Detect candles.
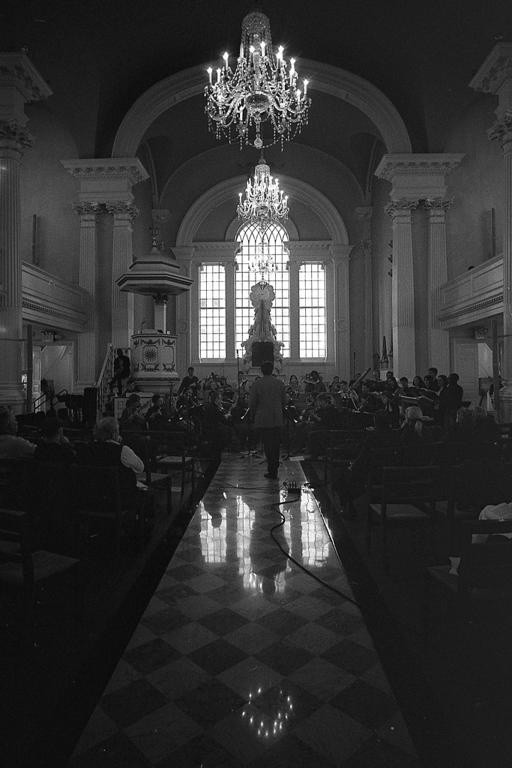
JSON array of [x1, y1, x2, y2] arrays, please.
[[235, 164, 290, 231]]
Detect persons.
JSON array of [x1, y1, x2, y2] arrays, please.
[[34, 416, 77, 469], [117, 363, 464, 478], [0, 403, 38, 458], [79, 415, 148, 492], [446, 485, 511, 584], [108, 348, 130, 393]]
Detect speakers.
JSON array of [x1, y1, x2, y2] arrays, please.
[[252, 344, 272, 367], [83, 386, 96, 426]]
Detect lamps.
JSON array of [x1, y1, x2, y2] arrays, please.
[[205, 12, 315, 151]]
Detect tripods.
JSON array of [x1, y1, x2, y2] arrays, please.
[[281, 418, 292, 461], [238, 415, 262, 459]]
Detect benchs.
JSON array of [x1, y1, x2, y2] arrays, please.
[[1, 419, 197, 732], [308, 425, 512, 682]]
